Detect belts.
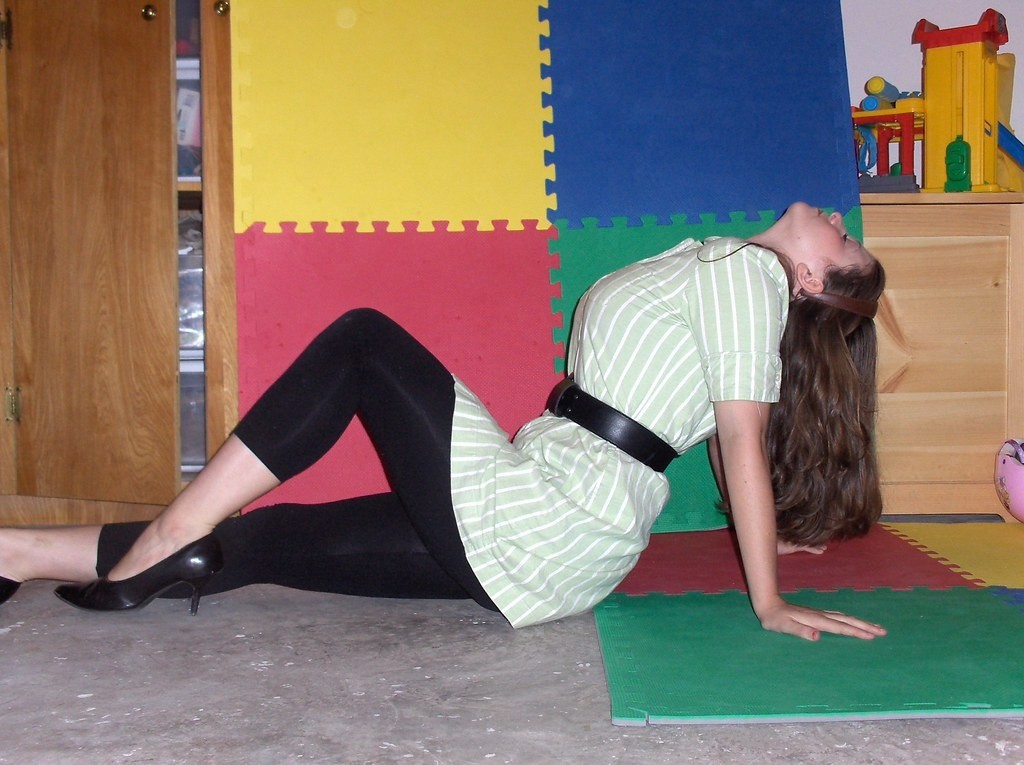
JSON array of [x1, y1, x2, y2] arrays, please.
[[548, 378, 681, 473]]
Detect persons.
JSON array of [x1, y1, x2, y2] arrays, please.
[[0, 200, 886, 640]]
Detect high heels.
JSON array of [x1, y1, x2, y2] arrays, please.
[[0, 576, 23, 605], [53, 533, 224, 616]]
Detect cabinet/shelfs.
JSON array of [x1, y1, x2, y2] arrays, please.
[[861, 192, 1024, 516]]
